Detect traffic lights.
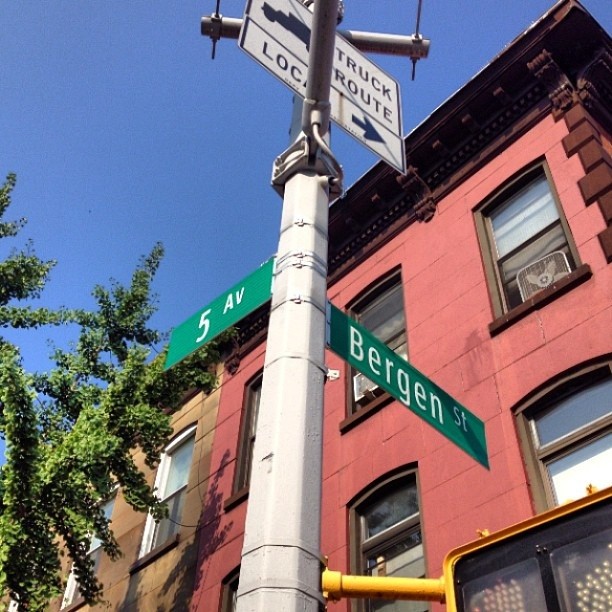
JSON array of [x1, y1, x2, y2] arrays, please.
[[319, 488, 612, 612]]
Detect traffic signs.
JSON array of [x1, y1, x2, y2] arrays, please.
[[163, 256, 274, 375], [237, 0, 409, 175], [325, 298, 491, 472]]
